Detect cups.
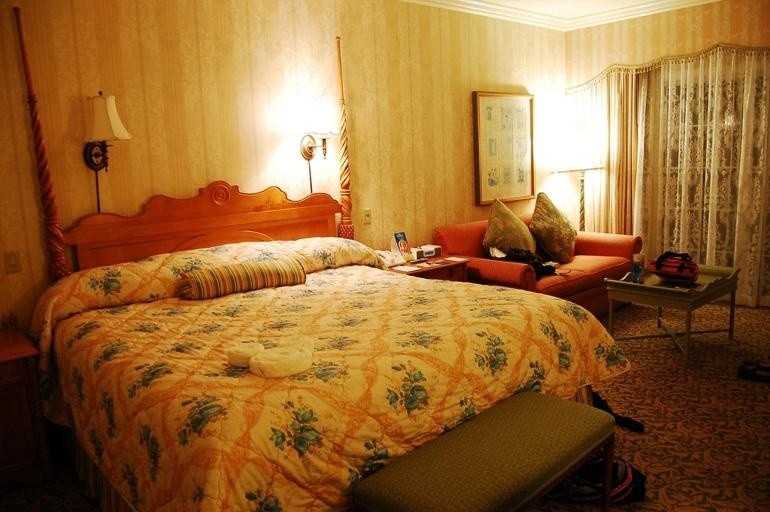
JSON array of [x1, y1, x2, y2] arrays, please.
[[632, 254, 646, 284]]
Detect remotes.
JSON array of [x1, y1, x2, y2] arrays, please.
[[410, 257, 428, 264]]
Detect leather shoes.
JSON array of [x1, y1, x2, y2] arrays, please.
[[738, 366, 770, 381], [744, 360, 770, 371]]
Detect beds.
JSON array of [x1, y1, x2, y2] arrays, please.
[[29, 178, 595, 511]]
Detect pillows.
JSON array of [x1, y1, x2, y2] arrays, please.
[[482, 191, 580, 265]]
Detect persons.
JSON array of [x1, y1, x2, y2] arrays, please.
[[399, 240, 410, 255]]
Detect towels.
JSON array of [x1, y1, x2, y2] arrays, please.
[[226, 334, 316, 379]]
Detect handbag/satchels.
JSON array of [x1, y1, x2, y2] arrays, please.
[[646, 251, 699, 286]]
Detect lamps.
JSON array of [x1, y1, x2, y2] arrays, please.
[[82, 89, 132, 173]]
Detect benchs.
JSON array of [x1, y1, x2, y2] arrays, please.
[[348, 388, 617, 511]]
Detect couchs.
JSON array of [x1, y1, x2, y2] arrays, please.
[[431, 212, 642, 317]]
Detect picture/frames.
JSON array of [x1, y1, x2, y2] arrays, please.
[[470, 90, 537, 207]]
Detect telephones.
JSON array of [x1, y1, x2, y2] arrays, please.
[[375, 250, 406, 268]]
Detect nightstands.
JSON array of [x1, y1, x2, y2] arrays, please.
[[383, 255, 471, 283], [0, 328, 50, 492]]
